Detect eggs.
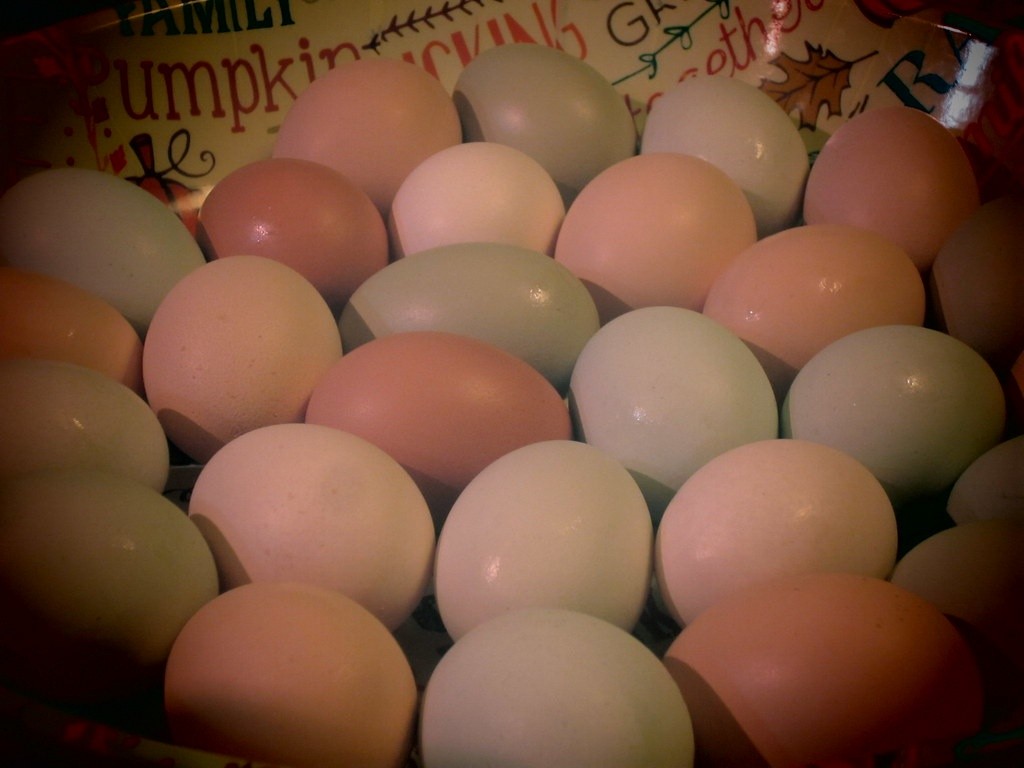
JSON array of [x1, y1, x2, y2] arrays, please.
[[1, 44, 1024, 768]]
